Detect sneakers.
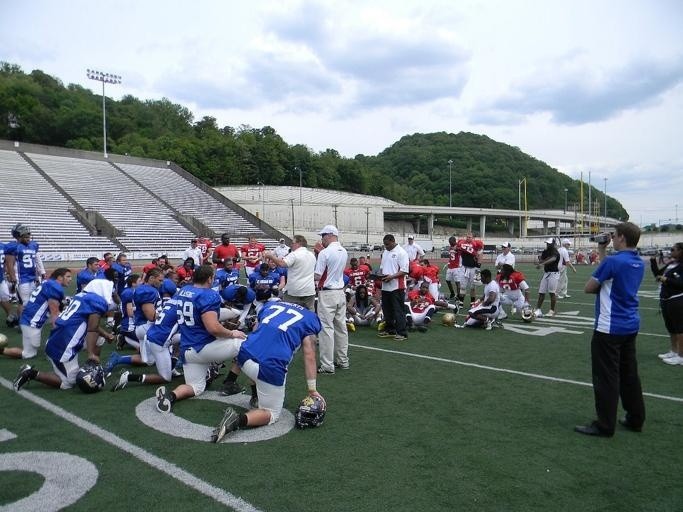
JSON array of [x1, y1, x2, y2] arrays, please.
[[657, 351, 683, 366]]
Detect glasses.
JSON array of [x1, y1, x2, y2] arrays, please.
[[670, 248, 679, 253]]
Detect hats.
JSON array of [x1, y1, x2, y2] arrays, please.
[[562, 239, 571, 244], [501, 242, 511, 248], [408, 236, 414, 239], [318, 225, 338, 236], [544, 238, 555, 245]]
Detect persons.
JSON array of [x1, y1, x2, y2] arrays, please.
[[572, 223, 646, 438], [648, 239, 683, 367], [572, 238, 666, 267], [350, 222, 572, 342], [1, 220, 349, 444]]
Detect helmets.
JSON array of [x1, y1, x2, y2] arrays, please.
[[473, 275, 483, 285], [12, 225, 30, 239]]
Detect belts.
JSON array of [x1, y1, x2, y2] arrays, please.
[[323, 287, 331, 291]]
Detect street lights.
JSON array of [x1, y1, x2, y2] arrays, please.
[[87, 68, 121, 158], [293, 166, 302, 206]]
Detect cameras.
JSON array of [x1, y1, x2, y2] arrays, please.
[[589, 232, 611, 243]]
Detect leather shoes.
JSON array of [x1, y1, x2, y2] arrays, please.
[[618, 419, 642, 433], [574, 425, 615, 437]]
